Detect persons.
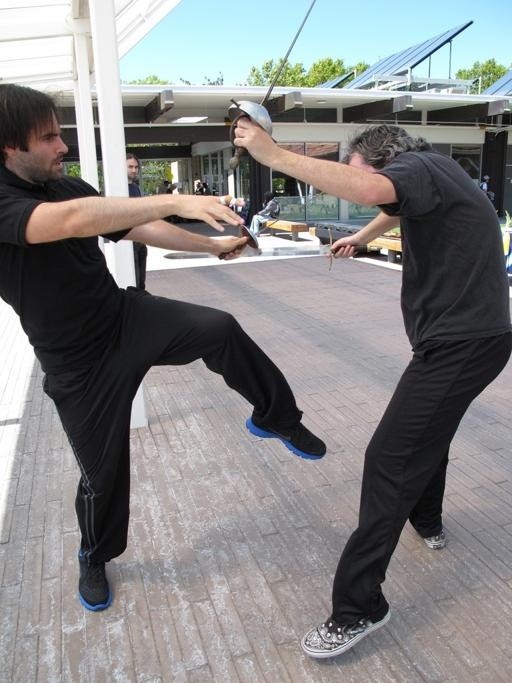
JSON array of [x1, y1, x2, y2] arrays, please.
[[479, 174, 490, 196], [126, 152, 147, 290], [194, 181, 202, 195], [233, 115, 509, 660], [0, 82, 329, 612], [202, 181, 210, 194], [250, 191, 280, 236], [158, 180, 169, 194]]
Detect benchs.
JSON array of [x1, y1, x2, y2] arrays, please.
[[263, 217, 404, 266]]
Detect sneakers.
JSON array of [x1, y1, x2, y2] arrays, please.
[[300, 598, 392, 659], [423, 528, 446, 550], [245, 411, 327, 461], [77, 547, 112, 611]]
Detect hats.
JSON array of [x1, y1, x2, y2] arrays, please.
[[483, 175, 490, 180]]
